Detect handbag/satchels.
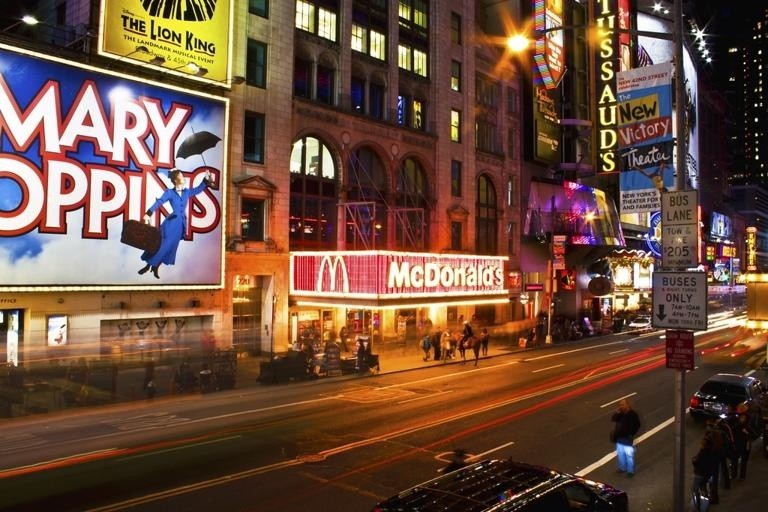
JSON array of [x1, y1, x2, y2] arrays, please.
[[120, 219, 162, 254], [609, 429, 617, 443]]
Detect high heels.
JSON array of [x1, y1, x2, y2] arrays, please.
[[139, 264, 150, 275], [150, 266, 160, 278]]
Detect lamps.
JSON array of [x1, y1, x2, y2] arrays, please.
[[3, 11, 38, 41], [107, 42, 247, 96]]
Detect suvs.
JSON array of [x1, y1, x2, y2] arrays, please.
[[370, 459, 628, 510]]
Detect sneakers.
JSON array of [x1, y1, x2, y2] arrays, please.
[[627, 470, 634, 477], [616, 467, 625, 473]]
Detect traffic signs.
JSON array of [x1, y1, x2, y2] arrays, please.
[[652, 270, 708, 331], [660, 190, 699, 269]]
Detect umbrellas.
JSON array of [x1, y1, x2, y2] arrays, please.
[[176, 130, 223, 159]]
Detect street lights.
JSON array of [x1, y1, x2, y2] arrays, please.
[[505, 1, 687, 509]]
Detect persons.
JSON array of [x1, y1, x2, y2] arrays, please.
[[609, 399, 641, 477], [301, 338, 314, 357], [690, 401, 751, 508], [419, 315, 490, 361], [138, 169, 210, 279], [552, 315, 584, 343], [357, 340, 365, 364], [319, 332, 340, 377], [339, 327, 349, 352]]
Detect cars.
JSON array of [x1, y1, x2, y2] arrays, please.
[[628, 315, 656, 333], [690, 372, 766, 425]]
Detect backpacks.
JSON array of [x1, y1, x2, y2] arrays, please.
[[418, 339, 425, 348]]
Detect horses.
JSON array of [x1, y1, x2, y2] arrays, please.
[[450, 331, 480, 366]]
